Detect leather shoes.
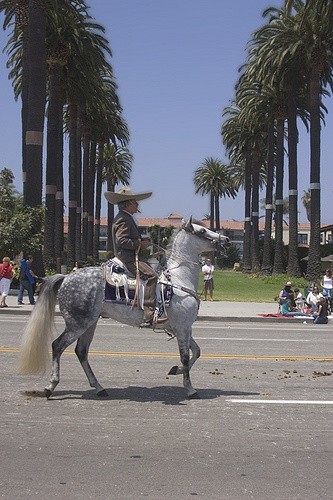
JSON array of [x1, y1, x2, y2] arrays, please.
[[142, 319, 169, 327]]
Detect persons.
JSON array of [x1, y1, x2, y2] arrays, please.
[[202, 259, 214, 301], [313, 295, 328, 324], [0, 257, 16, 308], [279, 282, 294, 305], [104, 187, 168, 327], [294, 288, 303, 313], [306, 286, 323, 312], [280, 293, 311, 317], [18, 255, 38, 305], [320, 269, 333, 312]]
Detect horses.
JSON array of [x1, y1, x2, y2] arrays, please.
[[16, 216, 230, 401]]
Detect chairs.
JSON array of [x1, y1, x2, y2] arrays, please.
[[274, 295, 282, 314]]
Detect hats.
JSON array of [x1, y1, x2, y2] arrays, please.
[[104, 187, 153, 205], [284, 281, 293, 287], [204, 259, 213, 262]]
